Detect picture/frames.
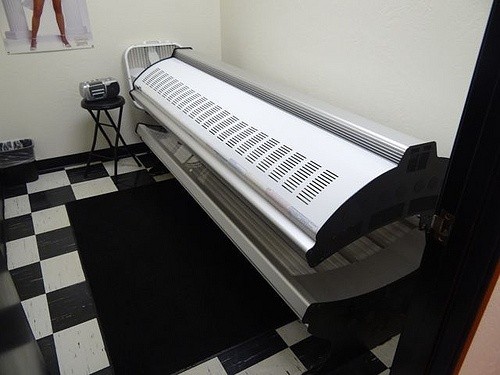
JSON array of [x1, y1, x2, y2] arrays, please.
[[1, 1, 95, 56]]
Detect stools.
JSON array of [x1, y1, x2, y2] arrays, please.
[[80, 95, 142, 177]]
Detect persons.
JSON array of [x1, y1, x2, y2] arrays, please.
[[29, 0, 71, 50]]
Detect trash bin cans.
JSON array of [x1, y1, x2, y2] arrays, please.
[[0, 138, 39, 186]]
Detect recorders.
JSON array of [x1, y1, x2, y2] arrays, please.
[[79, 77, 121, 101]]
